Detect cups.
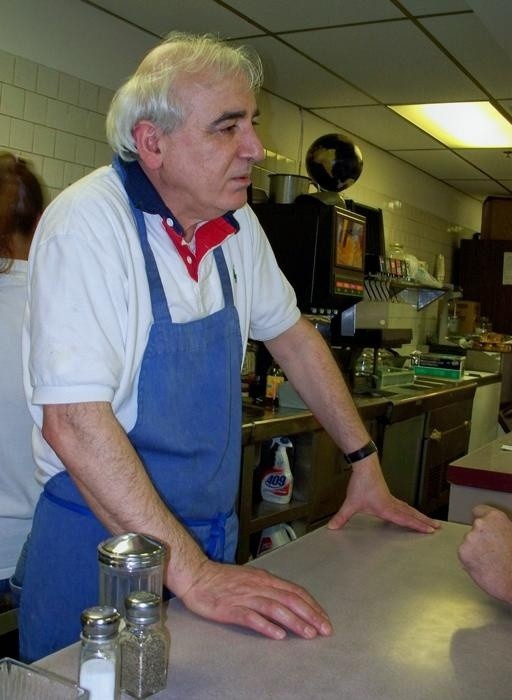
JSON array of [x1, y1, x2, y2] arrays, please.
[[266, 173, 321, 204]]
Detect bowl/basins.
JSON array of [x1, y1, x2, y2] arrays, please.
[[0, 657, 90, 700]]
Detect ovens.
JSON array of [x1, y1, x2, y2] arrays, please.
[[418, 394, 473, 519]]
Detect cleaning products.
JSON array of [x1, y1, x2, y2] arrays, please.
[[258, 436, 294, 504], [255, 523, 296, 560]]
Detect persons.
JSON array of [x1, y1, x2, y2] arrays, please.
[[458, 505, 512, 604], [15, 28, 443, 664], [2, 151, 43, 612]]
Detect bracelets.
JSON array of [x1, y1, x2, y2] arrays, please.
[[342, 439, 377, 465]]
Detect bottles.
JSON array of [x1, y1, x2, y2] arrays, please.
[[98, 532, 166, 629], [78, 605, 122, 700], [388, 242, 405, 261], [122, 592, 171, 700], [265, 360, 284, 408]]
